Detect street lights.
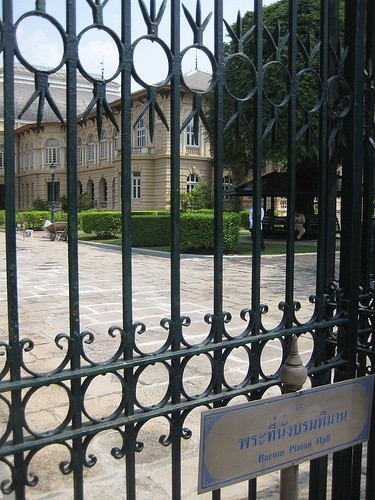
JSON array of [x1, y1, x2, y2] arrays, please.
[[49, 162, 57, 222]]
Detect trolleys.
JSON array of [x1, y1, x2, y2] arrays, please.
[[45, 222, 68, 242]]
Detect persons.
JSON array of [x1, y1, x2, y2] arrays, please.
[[294, 209, 306, 240], [249, 207, 271, 252]]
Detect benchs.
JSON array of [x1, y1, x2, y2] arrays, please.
[[262, 216, 341, 241]]
